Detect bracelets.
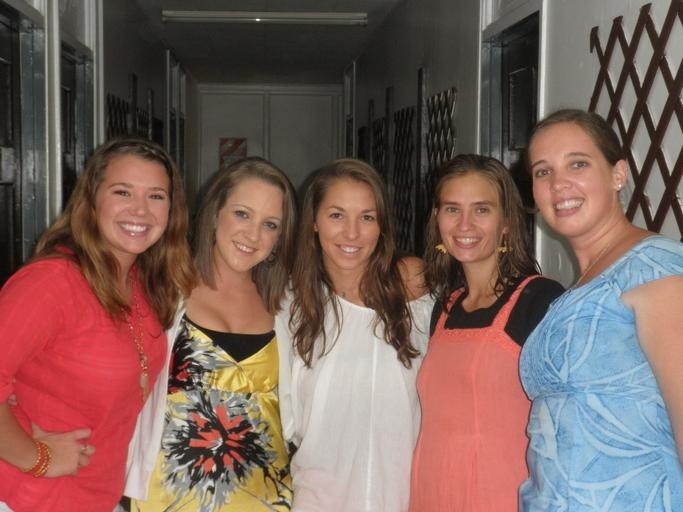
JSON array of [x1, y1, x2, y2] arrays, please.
[[22, 439, 54, 482]]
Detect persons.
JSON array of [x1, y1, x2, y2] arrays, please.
[[407, 152, 568, 512], [516, 109, 683, 512], [0, 136, 198, 512], [127, 155, 297, 512], [273, 159, 442, 512]]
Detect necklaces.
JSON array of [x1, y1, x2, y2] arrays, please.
[[571, 225, 632, 289], [103, 274, 155, 403]]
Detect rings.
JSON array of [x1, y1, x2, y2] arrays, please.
[[82, 442, 89, 453]]
